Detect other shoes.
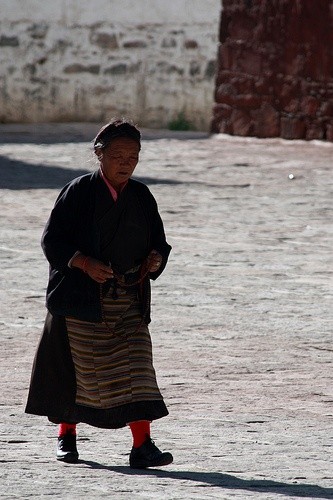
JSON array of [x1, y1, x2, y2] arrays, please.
[[129, 435, 173, 468], [57, 428, 79, 462]]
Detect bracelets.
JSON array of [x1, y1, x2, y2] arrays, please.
[[80, 256, 91, 273]]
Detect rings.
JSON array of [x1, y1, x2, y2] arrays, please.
[[154, 261, 160, 266]]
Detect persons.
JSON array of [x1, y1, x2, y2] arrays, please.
[[24, 118, 175, 469]]
